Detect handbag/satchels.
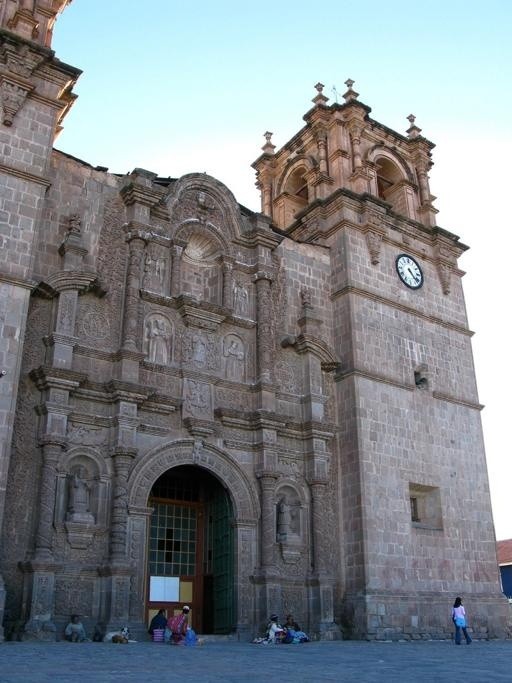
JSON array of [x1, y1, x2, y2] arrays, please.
[[455, 618, 466, 628], [153, 628, 164, 641]]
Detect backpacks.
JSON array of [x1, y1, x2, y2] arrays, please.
[[167, 615, 179, 630], [163, 625, 172, 644], [184, 630, 197, 646]]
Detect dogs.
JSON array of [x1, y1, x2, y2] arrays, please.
[[103, 626, 138, 643]]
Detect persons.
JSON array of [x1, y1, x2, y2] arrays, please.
[[278, 494, 296, 538], [63, 613, 87, 643], [144, 318, 169, 369], [223, 338, 244, 380], [68, 465, 97, 523], [265, 612, 286, 645], [147, 605, 169, 641], [232, 279, 250, 317], [143, 251, 167, 294], [451, 596, 473, 646], [282, 613, 310, 644], [172, 605, 196, 644]]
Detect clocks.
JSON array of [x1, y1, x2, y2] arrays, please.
[[396, 254, 425, 290]]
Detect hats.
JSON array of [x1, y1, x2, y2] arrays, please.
[[183, 605, 190, 610]]
